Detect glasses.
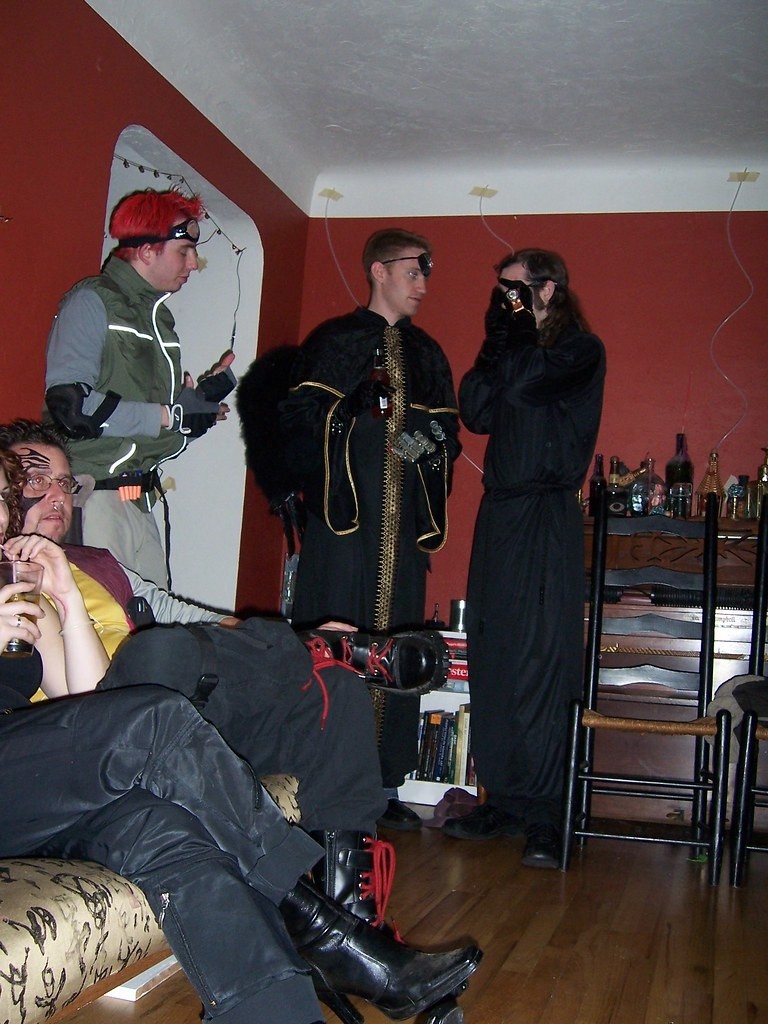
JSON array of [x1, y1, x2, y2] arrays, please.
[[119, 217, 200, 249], [27, 474, 80, 494]]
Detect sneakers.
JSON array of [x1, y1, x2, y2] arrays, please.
[[442, 804, 528, 841], [519, 820, 565, 869]]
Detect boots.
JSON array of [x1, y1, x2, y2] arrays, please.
[[310, 827, 405, 955], [301, 627, 452, 731], [279, 872, 484, 1024]]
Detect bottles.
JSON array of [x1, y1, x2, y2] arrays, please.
[[587, 431, 768, 519]]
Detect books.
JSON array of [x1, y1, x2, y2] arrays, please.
[[405, 704, 477, 787], [436, 630, 470, 694]]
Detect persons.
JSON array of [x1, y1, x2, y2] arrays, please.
[[0, 418, 484, 1024], [236, 228, 462, 830], [42, 186, 233, 594], [443, 243, 607, 872]]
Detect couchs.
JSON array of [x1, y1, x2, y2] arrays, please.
[[0, 686, 372, 1024]]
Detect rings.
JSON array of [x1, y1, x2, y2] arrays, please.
[[17, 618, 21, 627]]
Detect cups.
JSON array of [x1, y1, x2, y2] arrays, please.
[[449, 599, 467, 633], [0, 559, 45, 659]]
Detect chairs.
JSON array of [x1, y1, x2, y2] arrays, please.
[[559, 483, 731, 887], [729, 490, 768, 888]]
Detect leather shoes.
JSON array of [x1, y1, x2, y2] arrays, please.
[[376, 797, 423, 831]]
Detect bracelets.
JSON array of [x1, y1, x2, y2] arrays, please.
[[58, 620, 94, 635]]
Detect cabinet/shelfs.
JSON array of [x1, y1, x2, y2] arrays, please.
[[395, 631, 479, 806]]
